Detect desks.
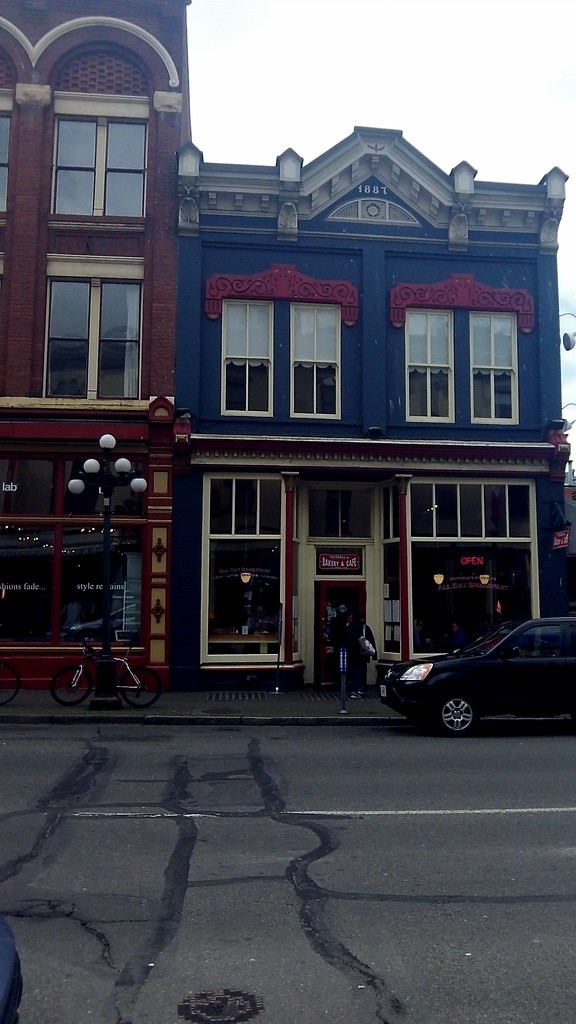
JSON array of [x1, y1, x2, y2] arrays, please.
[[208, 632, 280, 654]]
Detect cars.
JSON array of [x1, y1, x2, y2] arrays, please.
[[379, 612, 576, 737]]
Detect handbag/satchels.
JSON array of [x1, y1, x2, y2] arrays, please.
[[358, 624, 376, 657]]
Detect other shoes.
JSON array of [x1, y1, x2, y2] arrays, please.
[[351, 692, 361, 699], [358, 689, 367, 695]]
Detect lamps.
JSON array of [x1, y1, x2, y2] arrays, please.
[[433, 574, 445, 585], [240, 572, 252, 583], [395, 474, 413, 495], [281, 472, 300, 492], [480, 575, 490, 585], [552, 313, 576, 352]]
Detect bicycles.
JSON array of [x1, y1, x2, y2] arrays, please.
[[51, 619, 162, 709]]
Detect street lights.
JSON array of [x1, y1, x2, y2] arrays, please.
[[65, 430, 149, 711]]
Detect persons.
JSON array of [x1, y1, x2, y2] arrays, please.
[[414, 620, 434, 652], [347, 612, 378, 699], [445, 623, 471, 650], [323, 613, 353, 650]]
